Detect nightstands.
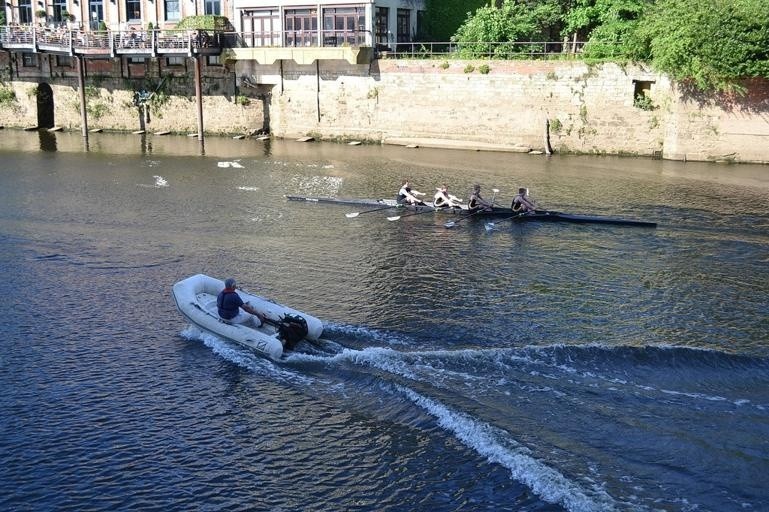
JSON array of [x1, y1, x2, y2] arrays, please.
[[344, 206, 393, 218], [485, 209, 536, 230], [387, 206, 440, 222], [444, 208, 486, 229]]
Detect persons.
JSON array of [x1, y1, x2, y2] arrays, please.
[[216, 278, 265, 328], [468, 184, 492, 211], [433, 184, 462, 207], [397, 180, 426, 206], [6, 22, 211, 48], [511, 187, 538, 213]]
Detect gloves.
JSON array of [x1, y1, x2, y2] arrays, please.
[[284, 193, 658, 228], [172, 273, 329, 366]]
